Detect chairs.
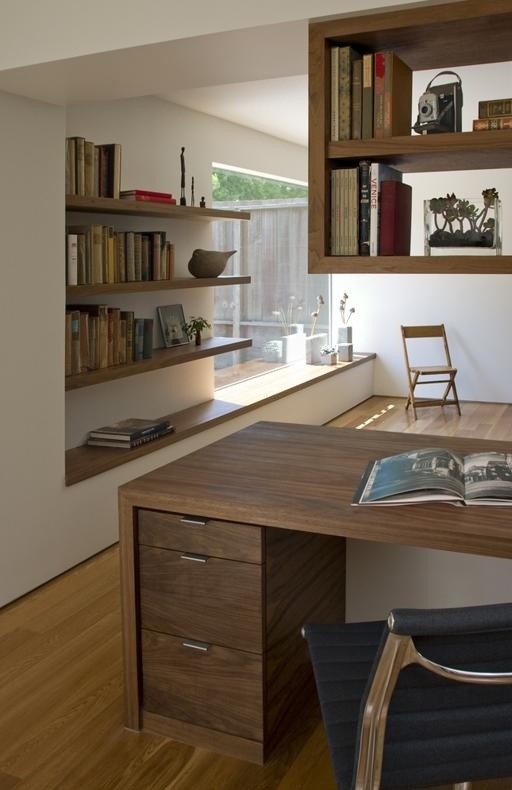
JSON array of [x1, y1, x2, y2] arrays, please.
[[304, 599, 511, 789], [400, 322, 463, 421]]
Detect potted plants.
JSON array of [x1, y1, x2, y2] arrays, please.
[[181, 316, 211, 347], [318, 344, 337, 365], [422, 189, 503, 256]]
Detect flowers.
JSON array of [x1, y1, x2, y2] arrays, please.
[[337, 292, 355, 329], [308, 294, 326, 334], [273, 295, 302, 335]]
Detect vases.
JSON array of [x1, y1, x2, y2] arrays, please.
[[305, 332, 328, 365], [336, 324, 354, 363], [279, 333, 304, 367]]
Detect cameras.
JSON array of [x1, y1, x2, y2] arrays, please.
[[417, 90, 440, 123]]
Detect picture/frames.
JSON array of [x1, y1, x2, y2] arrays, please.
[[156, 303, 190, 347]]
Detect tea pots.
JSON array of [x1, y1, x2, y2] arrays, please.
[[188, 249, 237, 278]]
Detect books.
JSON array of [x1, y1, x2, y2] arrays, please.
[[88, 416, 170, 443], [471, 114, 511, 130], [66, 136, 176, 379], [353, 448, 512, 508], [478, 97, 511, 116], [329, 47, 412, 256], [86, 424, 176, 449]]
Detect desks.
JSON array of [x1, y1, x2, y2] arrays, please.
[[116, 416, 512, 768]]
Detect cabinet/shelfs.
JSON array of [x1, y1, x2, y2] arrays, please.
[[66, 192, 252, 491], [306, 0, 512, 274]]
